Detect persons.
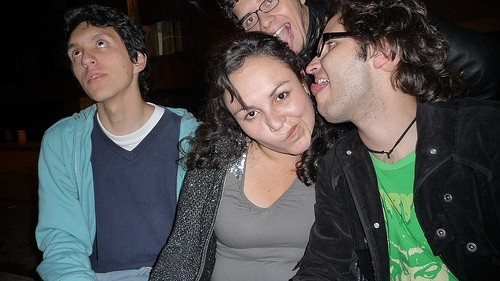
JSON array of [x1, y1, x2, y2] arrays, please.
[[219, 0, 500, 130], [288, 2, 500, 281], [35, 4, 203, 281], [149, 31, 347, 281]]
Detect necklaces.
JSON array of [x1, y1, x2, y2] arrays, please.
[[365, 118, 416, 165]]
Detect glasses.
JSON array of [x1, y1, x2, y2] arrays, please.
[[235, 0, 279, 31], [317, 32, 360, 58]]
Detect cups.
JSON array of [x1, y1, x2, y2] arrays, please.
[[17, 131, 25, 144]]
